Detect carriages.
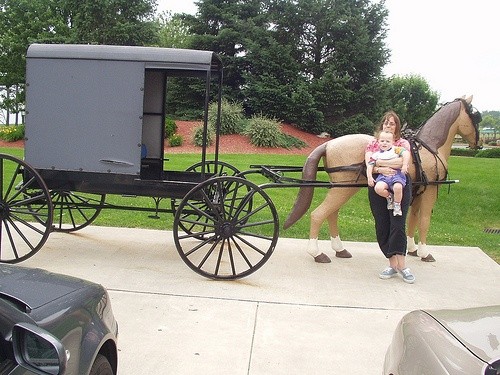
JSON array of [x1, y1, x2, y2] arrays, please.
[[0, 45, 484, 284]]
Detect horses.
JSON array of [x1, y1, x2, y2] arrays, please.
[[283, 92, 484, 264]]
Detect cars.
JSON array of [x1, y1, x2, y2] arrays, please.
[[383, 305, 499, 375], [0, 265, 120, 375]]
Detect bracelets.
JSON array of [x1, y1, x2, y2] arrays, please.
[[377, 168, 379, 172]]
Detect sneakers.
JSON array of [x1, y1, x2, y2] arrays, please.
[[386, 192, 393, 210], [397, 267, 415, 283], [379, 266, 398, 280], [393, 201, 402, 216]]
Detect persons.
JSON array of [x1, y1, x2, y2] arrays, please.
[[367, 130, 408, 217], [365, 112, 415, 284]]
